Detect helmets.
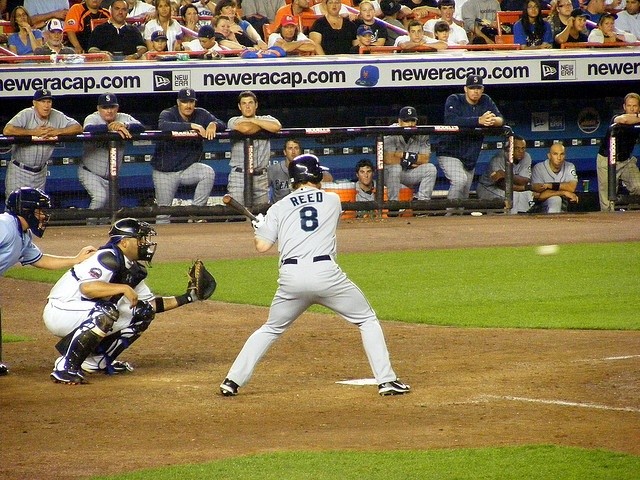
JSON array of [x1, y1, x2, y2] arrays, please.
[[288, 154, 330, 182], [5, 187, 50, 238], [110, 218, 157, 261]]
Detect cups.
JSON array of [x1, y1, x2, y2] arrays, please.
[[582, 179, 590, 192]]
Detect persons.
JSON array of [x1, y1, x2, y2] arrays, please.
[[219, 154, 410, 395], [241, 0, 287, 24], [268, 138, 333, 205], [76, 92, 146, 225], [596, 92, 640, 211], [275, 0, 319, 38], [42, 218, 200, 384], [582, 0, 607, 24], [179, 0, 216, 28], [461, 1, 502, 51], [553, 9, 590, 49], [512, 0, 553, 50], [423, 0, 469, 51], [571, 0, 590, 10], [384, 106, 437, 217], [143, 0, 182, 52], [108, 0, 155, 21], [3, 89, 83, 213], [309, 0, 357, 55], [174, 4, 202, 51], [268, 15, 316, 56], [588, 13, 637, 42], [63, 0, 111, 54], [356, 158, 387, 218], [351, 0, 388, 46], [434, 21, 461, 51], [349, 24, 376, 54], [310, 0, 350, 18], [141, 30, 168, 60], [212, 15, 256, 50], [531, 143, 579, 213], [398, 0, 437, 19], [436, 0, 468, 27], [436, 75, 504, 216], [23, 0, 70, 34], [604, 0, 627, 10], [614, 0, 640, 43], [0, 187, 97, 375], [546, 0, 573, 33], [33, 19, 76, 55], [214, 0, 268, 51], [7, 6, 42, 56], [476, 134, 535, 214], [393, 21, 449, 53], [151, 86, 226, 224], [88, 0, 147, 60], [380, 0, 409, 44], [183, 25, 223, 52], [227, 91, 280, 206]]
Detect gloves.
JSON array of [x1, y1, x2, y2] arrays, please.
[[251, 213, 265, 231]]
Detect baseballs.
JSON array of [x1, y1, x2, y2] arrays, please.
[[537, 244, 559, 255]]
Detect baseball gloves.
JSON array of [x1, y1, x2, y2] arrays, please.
[[187, 260, 217, 300]]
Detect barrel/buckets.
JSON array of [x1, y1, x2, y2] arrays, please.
[[322, 180, 357, 221], [374, 186, 414, 220]]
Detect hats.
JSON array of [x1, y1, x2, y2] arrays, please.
[[44, 19, 63, 31], [151, 30, 167, 40], [570, 8, 590, 20], [281, 15, 297, 26], [178, 88, 197, 101], [381, 0, 401, 15], [98, 93, 118, 106], [357, 24, 372, 35], [399, 107, 418, 122], [597, 12, 618, 23], [199, 26, 215, 38], [33, 90, 53, 99], [356, 66, 379, 85], [466, 75, 482, 88]]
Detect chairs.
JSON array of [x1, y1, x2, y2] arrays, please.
[[414, 11, 438, 22], [0, 23, 22, 36], [261, 24, 279, 42], [91, 19, 109, 32], [497, 10, 523, 44], [299, 13, 323, 27]]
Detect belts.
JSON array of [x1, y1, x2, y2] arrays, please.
[[234, 166, 266, 175], [283, 255, 330, 263], [13, 160, 46, 173], [83, 166, 110, 182]]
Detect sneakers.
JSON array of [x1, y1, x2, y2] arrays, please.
[[81, 354, 132, 372], [187, 219, 207, 222], [0, 363, 8, 376], [379, 378, 410, 395], [50, 357, 84, 384], [220, 378, 238, 395]]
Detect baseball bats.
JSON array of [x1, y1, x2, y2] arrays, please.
[[222, 194, 258, 222]]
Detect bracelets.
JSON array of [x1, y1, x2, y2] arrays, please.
[[551, 183, 560, 191], [403, 151, 418, 163], [401, 159, 413, 170]]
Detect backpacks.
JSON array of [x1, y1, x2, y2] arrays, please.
[[473, 27, 497, 51]]
